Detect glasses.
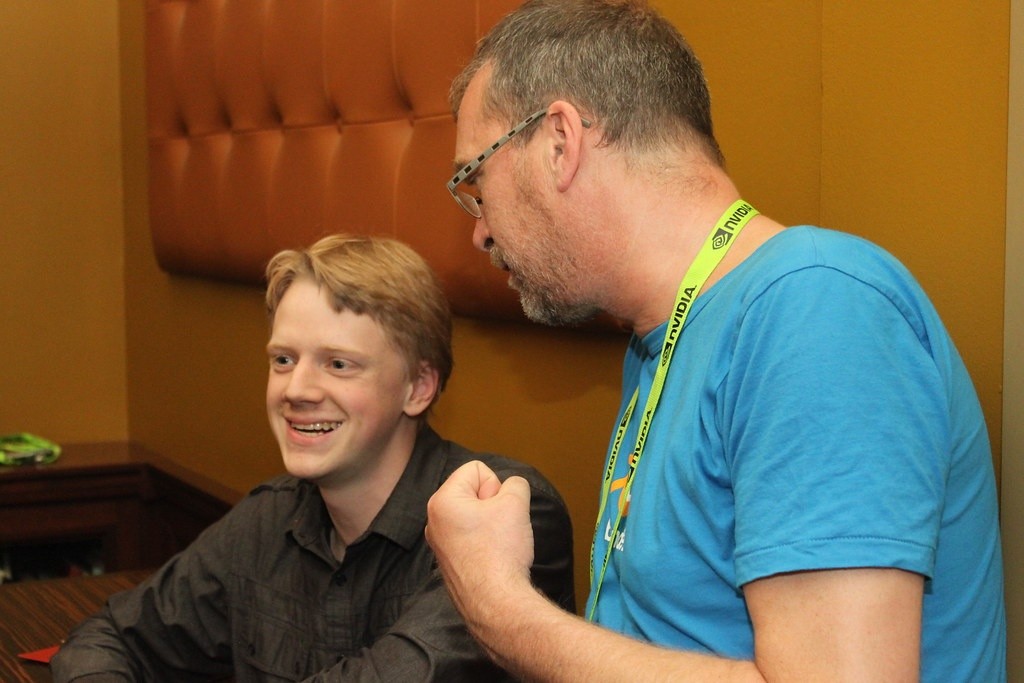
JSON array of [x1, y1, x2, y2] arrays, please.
[[445, 109, 592, 218]]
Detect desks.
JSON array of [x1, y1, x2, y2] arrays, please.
[[0, 567, 158, 683], [0, 437, 148, 569]]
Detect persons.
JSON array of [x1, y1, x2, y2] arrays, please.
[[55, 235, 577, 683], [424, 0, 1007, 683]]
